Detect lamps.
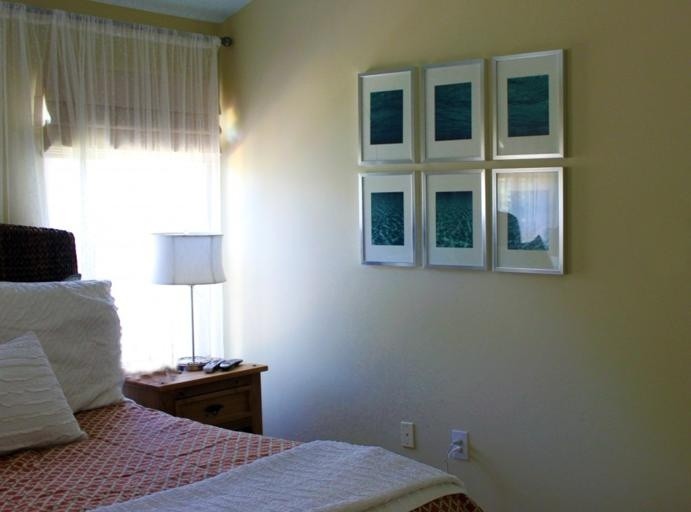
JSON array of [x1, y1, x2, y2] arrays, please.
[[151, 233, 226, 369]]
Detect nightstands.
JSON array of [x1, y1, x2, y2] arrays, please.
[[125, 361, 268, 436]]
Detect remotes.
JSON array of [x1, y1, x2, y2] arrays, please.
[[221, 359, 243, 370], [203, 358, 225, 372]]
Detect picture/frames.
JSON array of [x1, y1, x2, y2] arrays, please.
[[359, 171, 416, 268], [491, 48, 565, 159], [492, 167, 564, 275], [420, 59, 485, 164], [421, 168, 488, 271], [357, 69, 415, 162]]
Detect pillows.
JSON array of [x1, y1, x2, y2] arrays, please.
[[0, 278, 125, 412], [0, 331, 87, 451]]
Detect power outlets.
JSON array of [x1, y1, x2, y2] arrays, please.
[[401, 420, 414, 448], [452, 429, 468, 460]]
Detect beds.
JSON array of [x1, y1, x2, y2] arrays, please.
[[1, 222, 479, 511]]
